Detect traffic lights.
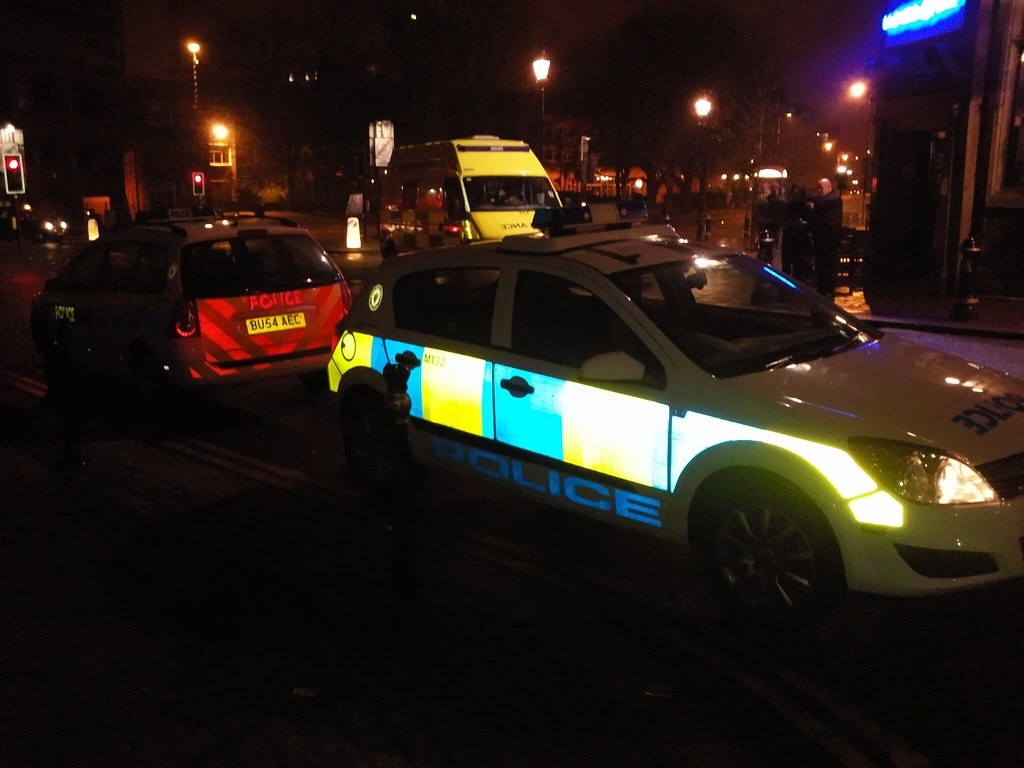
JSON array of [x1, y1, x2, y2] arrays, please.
[[3, 154, 26, 195], [191, 171, 206, 197]]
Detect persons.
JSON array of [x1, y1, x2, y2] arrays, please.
[[760, 180, 784, 265], [808, 177, 842, 301], [780, 185, 812, 279]]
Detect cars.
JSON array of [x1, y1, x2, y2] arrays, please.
[[322, 206, 1024, 647], [617, 198, 649, 222], [27, 210, 351, 410]]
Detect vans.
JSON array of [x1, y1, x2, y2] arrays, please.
[[368, 135, 565, 256]]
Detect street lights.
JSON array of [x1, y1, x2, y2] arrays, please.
[[187, 40, 201, 115], [530, 50, 553, 163], [690, 93, 716, 242]]
